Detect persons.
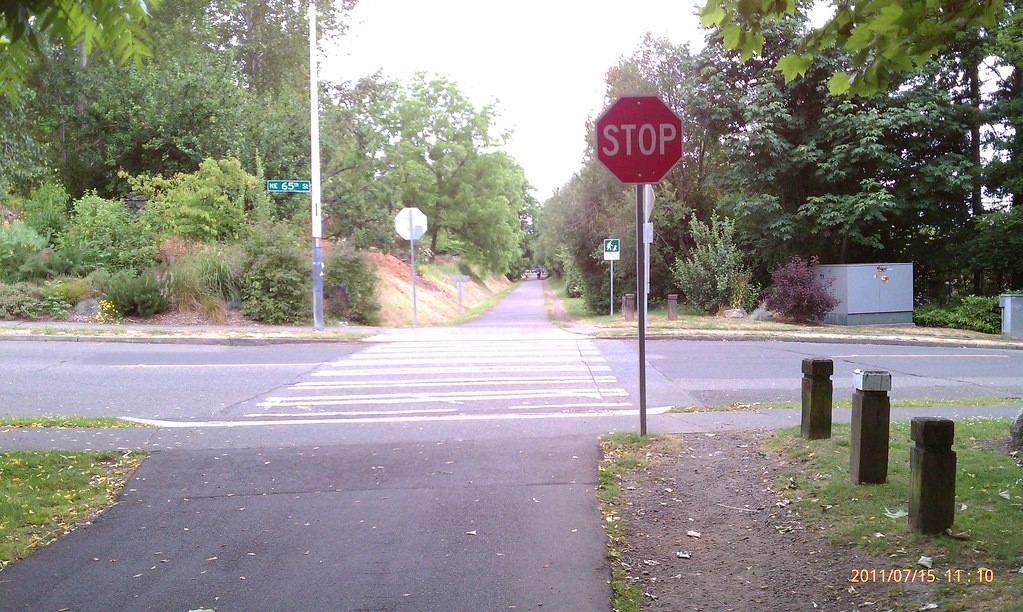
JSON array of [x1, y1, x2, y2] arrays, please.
[[536, 267, 541, 279]]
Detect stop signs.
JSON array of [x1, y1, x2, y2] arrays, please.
[[593, 94, 684, 185]]
